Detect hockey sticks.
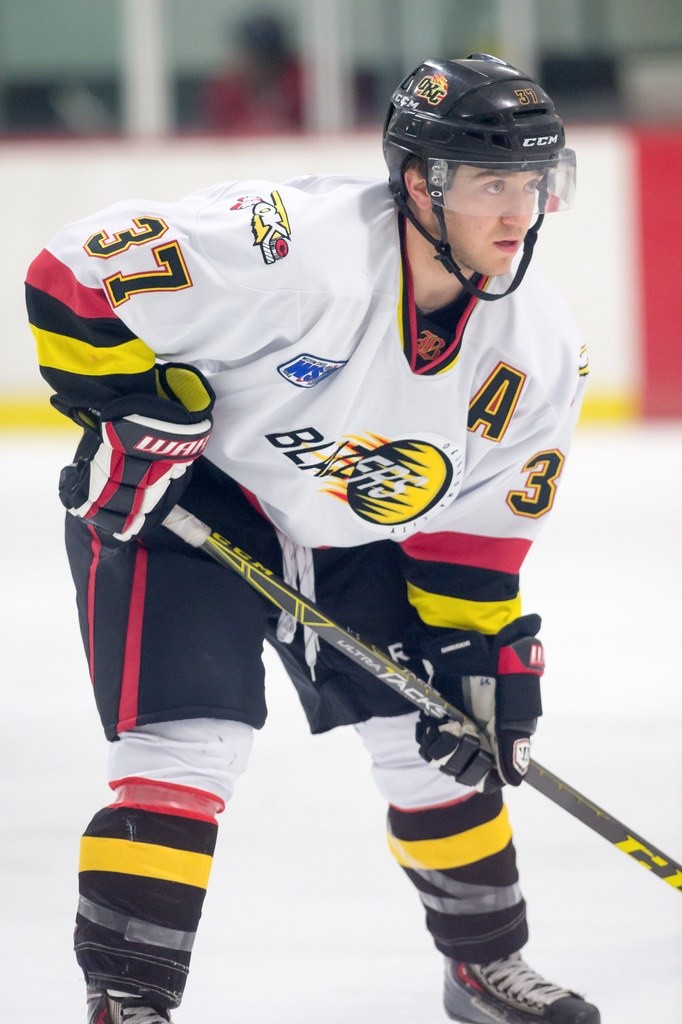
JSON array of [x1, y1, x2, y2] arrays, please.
[[59, 457, 682, 896]]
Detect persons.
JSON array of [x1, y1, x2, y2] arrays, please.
[[22, 56, 604, 1024]]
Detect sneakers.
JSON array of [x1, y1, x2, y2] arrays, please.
[[88, 985, 173, 1023], [444, 949, 601, 1024]]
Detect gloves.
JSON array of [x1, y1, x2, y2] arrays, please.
[[418, 611, 546, 793], [59, 395, 220, 545]]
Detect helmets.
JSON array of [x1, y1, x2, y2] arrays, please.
[[381, 51, 565, 202]]
